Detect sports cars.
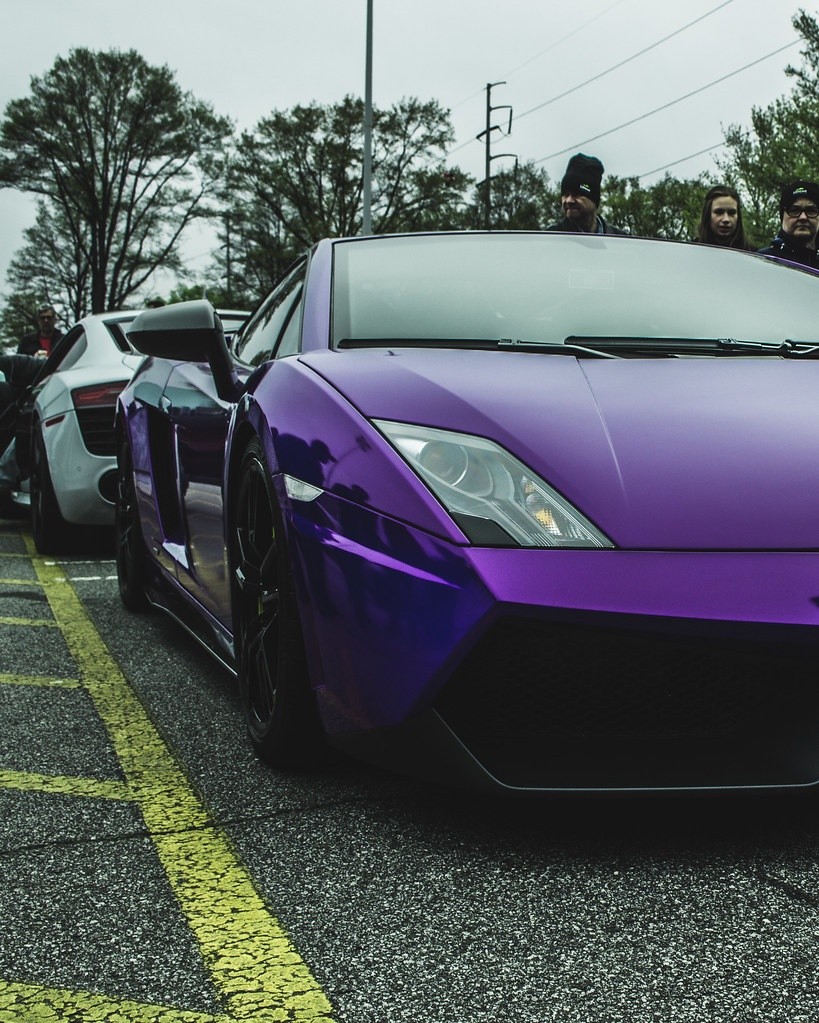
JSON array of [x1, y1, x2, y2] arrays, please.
[[112, 231, 819, 804]]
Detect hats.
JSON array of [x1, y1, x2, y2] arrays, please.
[[780, 181, 819, 221], [560, 153, 604, 208]]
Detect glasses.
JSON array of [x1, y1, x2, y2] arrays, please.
[[782, 203, 819, 218], [39, 315, 54, 321]]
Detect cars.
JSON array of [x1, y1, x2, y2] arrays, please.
[[0, 307, 259, 559]]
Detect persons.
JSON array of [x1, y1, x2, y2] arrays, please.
[[546, 153, 626, 234], [692, 185, 757, 253], [757, 182, 819, 270], [17, 304, 63, 359]]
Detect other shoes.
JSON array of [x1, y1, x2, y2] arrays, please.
[[0, 488, 32, 520]]
